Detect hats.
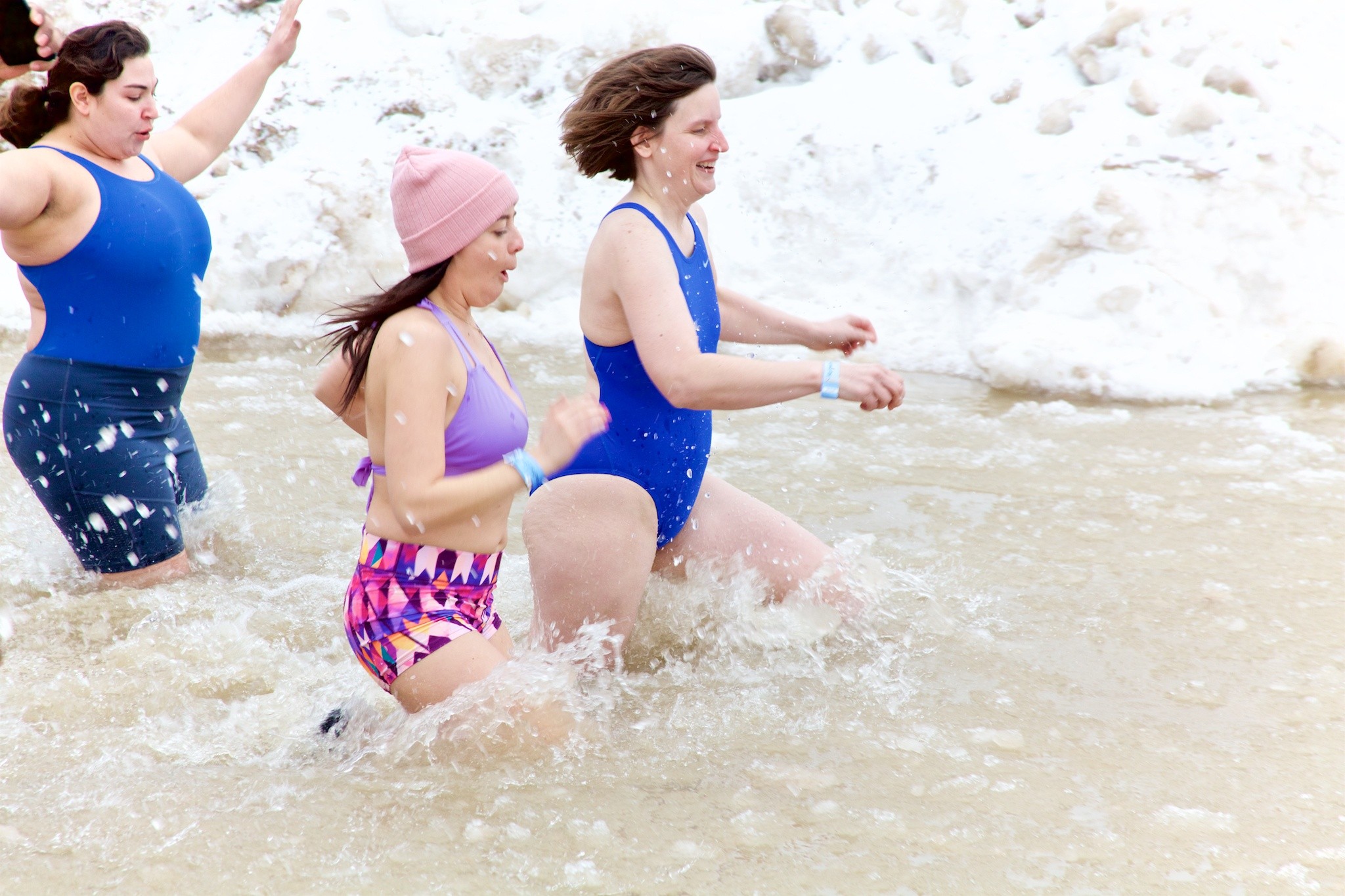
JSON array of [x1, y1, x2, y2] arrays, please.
[[386, 144, 518, 274]]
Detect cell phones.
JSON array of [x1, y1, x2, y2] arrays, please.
[[0, 0, 55, 66]]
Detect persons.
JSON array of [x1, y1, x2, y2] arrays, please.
[[0, 0, 303, 586], [0, 2, 67, 87], [314, 145, 610, 714], [522, 42, 904, 675]]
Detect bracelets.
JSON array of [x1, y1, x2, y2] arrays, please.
[[505, 448, 545, 492], [820, 361, 840, 398]]
